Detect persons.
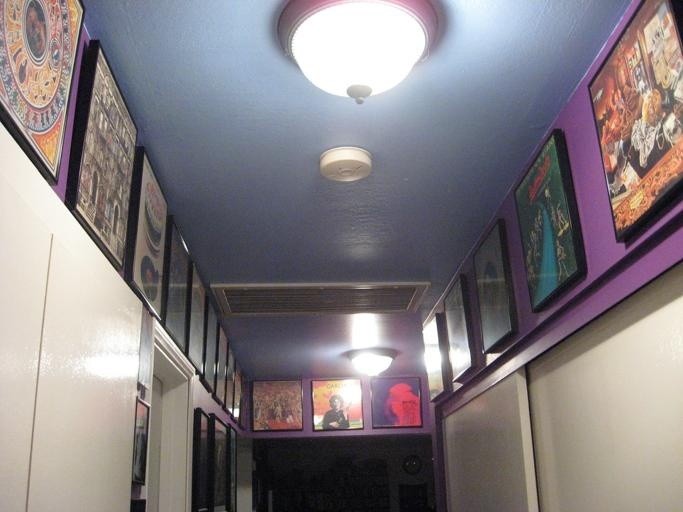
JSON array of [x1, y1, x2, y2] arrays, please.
[[322, 394, 350, 429]]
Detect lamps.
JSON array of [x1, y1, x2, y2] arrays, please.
[[276, 1, 439, 105], [317, 146, 373, 183], [345, 348, 397, 377]]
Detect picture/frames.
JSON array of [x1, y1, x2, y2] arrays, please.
[[65, 39, 139, 272], [223, 344, 235, 416], [230, 366, 246, 431], [469, 217, 519, 363], [512, 127, 588, 314], [198, 294, 220, 395], [585, 1, 682, 247], [121, 144, 168, 321], [442, 265, 477, 387], [164, 213, 193, 356], [193, 405, 236, 511], [131, 394, 150, 486], [3, 2, 86, 188], [421, 312, 450, 404], [250, 375, 423, 432], [211, 322, 230, 407], [187, 261, 210, 376]]
